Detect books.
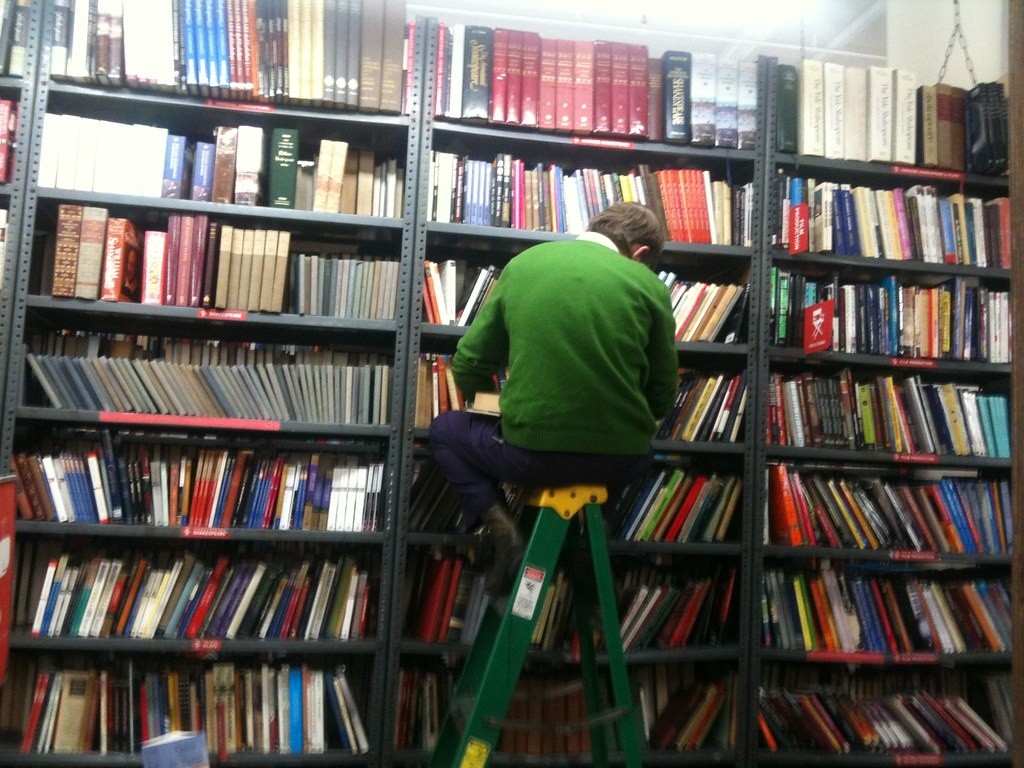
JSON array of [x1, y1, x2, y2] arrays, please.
[[1, 1, 1011, 768]]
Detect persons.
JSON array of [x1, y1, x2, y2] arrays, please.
[[427, 201, 678, 598]]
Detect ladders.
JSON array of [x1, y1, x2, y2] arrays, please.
[[429, 486, 643, 768]]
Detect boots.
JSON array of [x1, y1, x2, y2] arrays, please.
[[480, 504, 527, 594]]
